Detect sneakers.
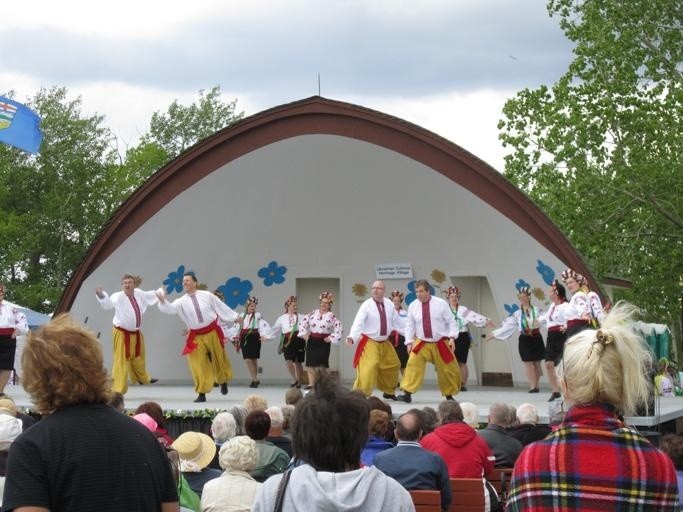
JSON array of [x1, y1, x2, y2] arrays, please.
[[528, 387, 539, 393], [291, 380, 311, 389], [446, 387, 467, 400], [193, 393, 205, 402], [221, 383, 227, 394], [250, 380, 260, 388], [138, 377, 158, 384], [383, 392, 411, 402], [548, 392, 561, 401]]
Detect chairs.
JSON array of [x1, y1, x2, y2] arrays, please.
[[407, 466, 513, 512]]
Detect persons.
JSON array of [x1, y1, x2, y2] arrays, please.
[[485, 278, 546, 392], [505, 298, 681, 512], [297, 289, 343, 390], [1, 397, 32, 448], [95, 273, 165, 399], [389, 288, 408, 389], [535, 278, 569, 405], [0, 282, 29, 397], [229, 296, 271, 388], [258, 295, 308, 389], [359, 397, 550, 512], [153, 272, 245, 405], [390, 279, 462, 403], [346, 279, 408, 400], [446, 285, 497, 391], [109, 388, 303, 511], [207, 289, 225, 387], [579, 274, 606, 331], [560, 267, 591, 340], [0, 313, 181, 511], [655, 357, 683, 510]]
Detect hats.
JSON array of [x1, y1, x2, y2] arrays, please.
[[169, 431, 217, 473], [132, 412, 157, 432]]
